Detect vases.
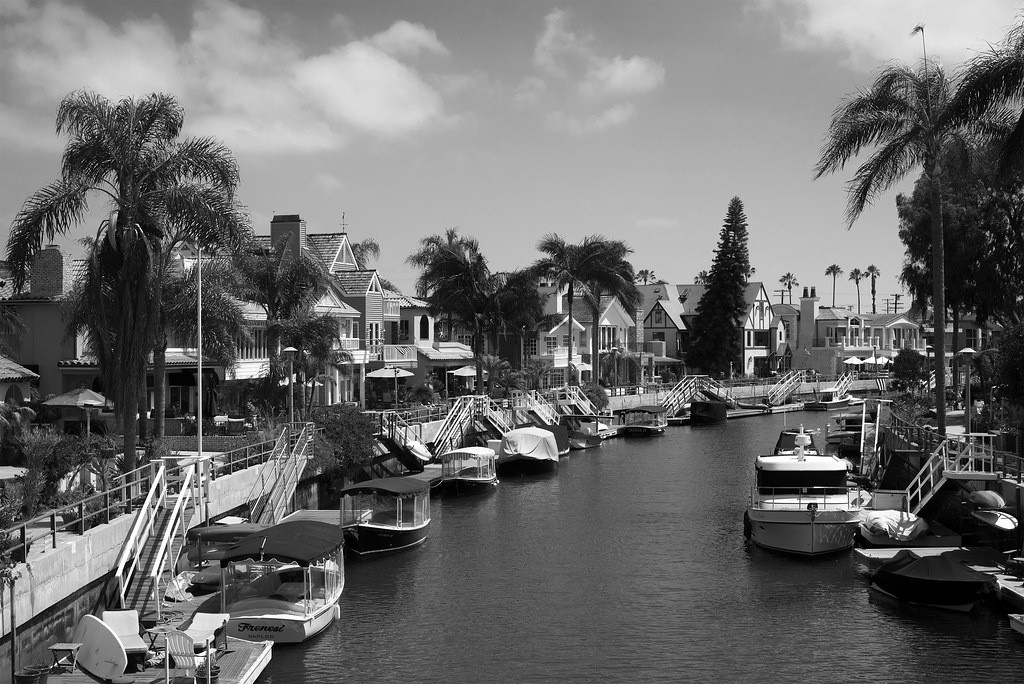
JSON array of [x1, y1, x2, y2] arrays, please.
[[14, 670, 41, 684], [24, 663, 49, 684]]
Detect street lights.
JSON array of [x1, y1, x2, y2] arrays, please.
[[282, 345, 298, 431]]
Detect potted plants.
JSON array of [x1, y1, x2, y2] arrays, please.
[[223, 432, 273, 475], [194, 653, 221, 684], [0, 424, 183, 569]]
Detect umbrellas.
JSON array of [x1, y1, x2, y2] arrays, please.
[[447, 365, 488, 377], [367, 365, 414, 411], [41, 383, 117, 438]]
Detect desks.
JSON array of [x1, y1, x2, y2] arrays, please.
[[1013, 558, 1024, 580], [47, 643, 84, 674], [276, 581, 314, 600], [144, 627, 174, 651]]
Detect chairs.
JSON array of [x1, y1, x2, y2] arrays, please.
[[164, 630, 211, 684], [1004, 550, 1022, 576], [183, 612, 230, 657], [101, 608, 149, 672]]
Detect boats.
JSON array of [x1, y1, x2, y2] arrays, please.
[[858, 486, 1024, 636], [617, 405, 669, 437], [824, 410, 875, 452], [538, 424, 573, 456], [330, 474, 434, 556], [159, 517, 347, 647], [497, 426, 559, 475], [440, 446, 500, 499], [742, 420, 858, 554], [801, 393, 853, 411], [557, 411, 616, 449], [865, 547, 1000, 618]]
